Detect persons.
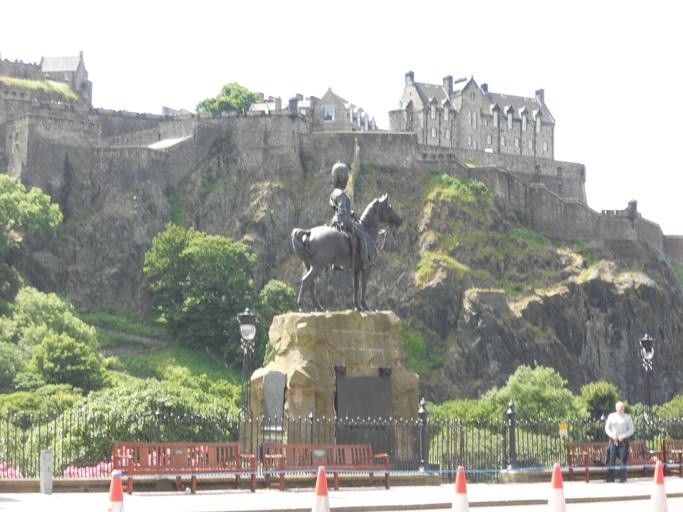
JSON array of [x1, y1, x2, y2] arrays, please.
[[604, 400, 634, 483], [329, 163, 377, 270]]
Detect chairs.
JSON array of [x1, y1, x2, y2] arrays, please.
[[565, 441, 661, 482], [262, 442, 390, 490], [112, 441, 256, 494], [662, 440, 683, 477]]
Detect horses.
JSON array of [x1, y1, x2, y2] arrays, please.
[[290, 192, 403, 313]]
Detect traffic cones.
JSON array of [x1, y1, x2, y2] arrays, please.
[[451, 466, 471, 512], [648, 461, 669, 512], [106, 470, 123, 512], [310, 466, 329, 512], [545, 462, 565, 512]]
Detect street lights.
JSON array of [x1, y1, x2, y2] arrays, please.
[[639, 333, 655, 456], [235, 307, 257, 469]]
